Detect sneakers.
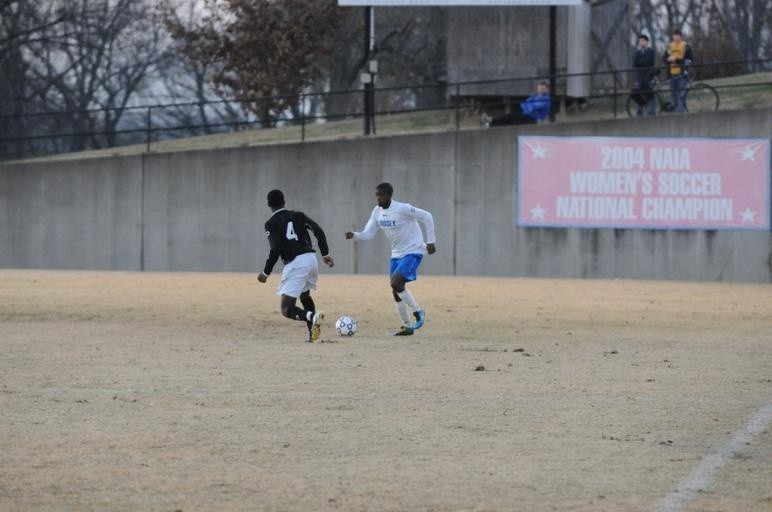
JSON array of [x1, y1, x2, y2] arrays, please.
[[413, 309, 425, 329], [309, 310, 324, 341], [393, 326, 413, 335]]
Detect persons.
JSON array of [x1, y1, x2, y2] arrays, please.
[[345, 182, 437, 336], [662, 30, 694, 113], [632, 36, 656, 115], [480, 81, 552, 126], [257, 189, 334, 344]]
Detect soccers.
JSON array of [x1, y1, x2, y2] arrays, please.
[[336, 316, 356, 336]]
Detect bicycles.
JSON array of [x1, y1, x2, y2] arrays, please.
[[627, 61, 720, 116]]
